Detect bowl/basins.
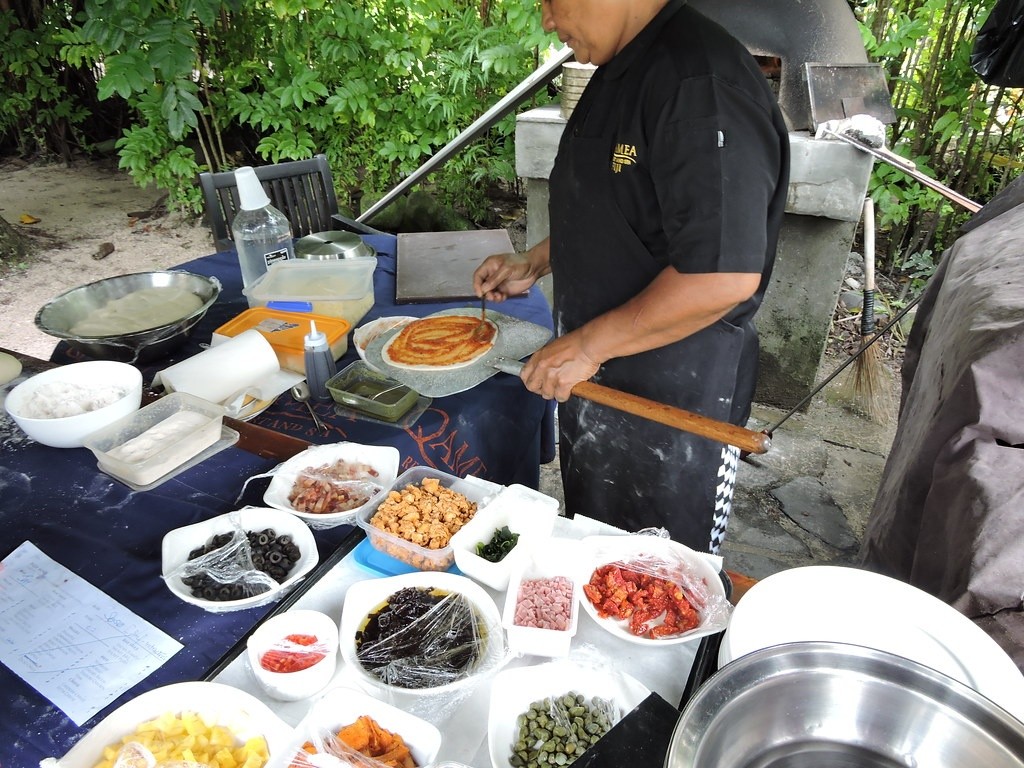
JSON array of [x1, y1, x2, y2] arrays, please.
[[213, 306, 351, 376], [665, 641, 1023, 768], [247, 609, 338, 701], [501, 566, 582, 659], [448, 483, 560, 592], [353, 316, 419, 374], [242, 257, 377, 334], [81, 392, 240, 492], [35, 271, 220, 364], [355, 466, 495, 572], [4, 360, 143, 448], [263, 689, 442, 768], [324, 360, 433, 429]]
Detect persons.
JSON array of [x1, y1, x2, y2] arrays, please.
[[473, 0, 792, 546]]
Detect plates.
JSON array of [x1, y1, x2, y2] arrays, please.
[[263, 443, 400, 524], [162, 507, 319, 613], [486, 662, 652, 768], [52, 681, 294, 768], [339, 570, 502, 694], [237, 395, 278, 422], [574, 535, 728, 646], [716, 566, 1024, 721]]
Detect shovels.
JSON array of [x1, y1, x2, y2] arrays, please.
[[364, 308, 772, 455]]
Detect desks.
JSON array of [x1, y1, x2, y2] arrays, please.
[[0, 234, 1024, 768]]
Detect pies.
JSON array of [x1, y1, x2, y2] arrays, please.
[[381, 316, 499, 372]]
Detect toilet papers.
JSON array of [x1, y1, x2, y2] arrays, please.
[[149, 328, 281, 406]]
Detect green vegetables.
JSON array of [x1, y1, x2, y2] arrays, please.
[[476, 525, 519, 562]]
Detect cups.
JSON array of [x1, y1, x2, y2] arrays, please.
[[234, 166, 271, 211]]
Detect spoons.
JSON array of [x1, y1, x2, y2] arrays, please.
[[473, 281, 487, 342], [291, 381, 328, 436]]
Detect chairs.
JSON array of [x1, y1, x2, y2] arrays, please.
[[199, 152, 397, 254]]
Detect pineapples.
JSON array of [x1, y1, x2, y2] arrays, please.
[[88, 711, 270, 768]]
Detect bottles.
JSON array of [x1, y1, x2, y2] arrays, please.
[[304, 321, 339, 404], [231, 204, 296, 309]]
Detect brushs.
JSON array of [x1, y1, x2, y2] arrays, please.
[[841, 114, 916, 171]]
